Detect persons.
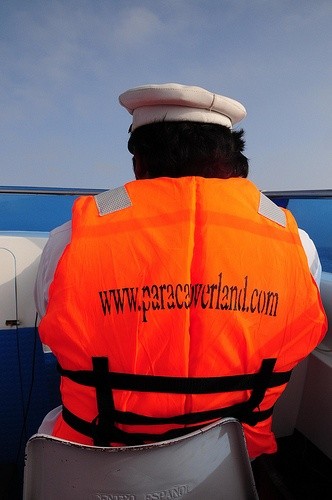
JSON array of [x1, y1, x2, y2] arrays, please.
[[34, 82, 328, 459]]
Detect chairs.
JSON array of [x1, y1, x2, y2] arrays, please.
[[23, 416, 259, 500]]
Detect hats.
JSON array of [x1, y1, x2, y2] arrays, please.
[[118, 82, 246, 132]]
